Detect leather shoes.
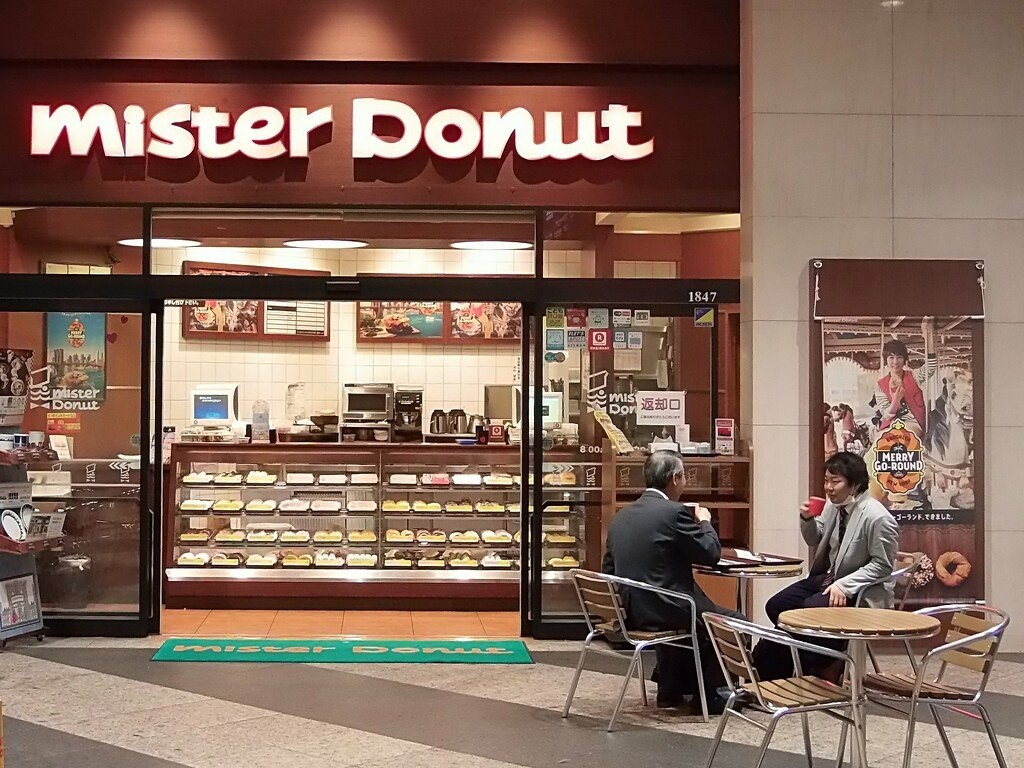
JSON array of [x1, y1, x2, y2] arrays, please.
[[654, 685, 756, 711]]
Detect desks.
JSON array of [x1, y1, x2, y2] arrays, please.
[[691, 563, 804, 687], [778, 606, 942, 767]]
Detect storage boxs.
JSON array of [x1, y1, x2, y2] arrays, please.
[[0, 528, 68, 556]]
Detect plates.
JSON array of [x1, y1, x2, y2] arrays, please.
[[2, 509, 26, 541], [455, 437, 477, 445], [19, 504, 36, 531]]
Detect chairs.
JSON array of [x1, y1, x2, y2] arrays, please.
[[842, 603, 1008, 768], [702, 610, 870, 767], [561, 568, 710, 733], [855, 552, 921, 672]]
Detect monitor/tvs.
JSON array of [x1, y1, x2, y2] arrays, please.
[[190, 385, 240, 426], [512, 386, 562, 431]]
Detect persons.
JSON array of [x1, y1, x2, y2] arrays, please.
[[601, 448, 750, 714], [876, 321, 937, 441], [833, 403, 855, 420], [766, 452, 901, 684]]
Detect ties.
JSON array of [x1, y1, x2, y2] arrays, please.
[[821, 505, 847, 593]]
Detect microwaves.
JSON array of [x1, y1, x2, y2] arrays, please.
[[340, 379, 394, 421]]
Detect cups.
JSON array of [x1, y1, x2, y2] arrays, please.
[[683, 502, 699, 520], [807, 495, 826, 516]]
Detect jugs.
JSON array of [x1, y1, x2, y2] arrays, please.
[[448, 409, 466, 434], [467, 415, 490, 433], [431, 409, 446, 434]]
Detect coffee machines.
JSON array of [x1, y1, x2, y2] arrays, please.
[[393, 383, 425, 444]]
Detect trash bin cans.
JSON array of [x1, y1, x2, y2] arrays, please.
[[57, 554, 93, 611]]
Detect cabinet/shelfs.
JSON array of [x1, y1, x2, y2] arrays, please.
[[160, 442, 602, 610]]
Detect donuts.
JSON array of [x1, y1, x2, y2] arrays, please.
[[936, 550, 972, 586], [903, 552, 934, 589]]
